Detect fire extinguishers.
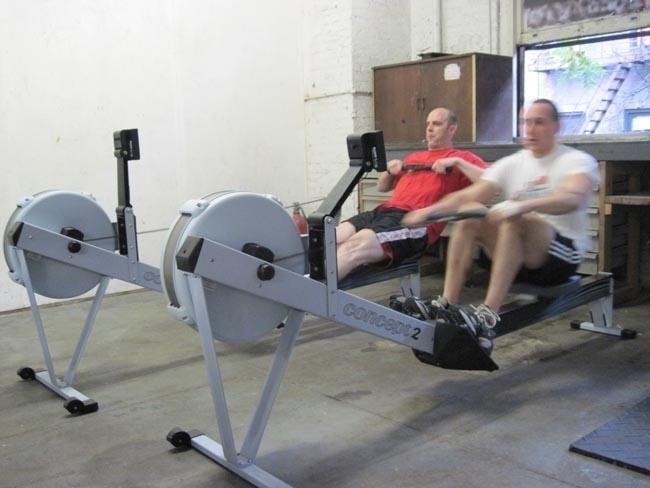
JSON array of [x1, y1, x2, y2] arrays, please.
[[292, 202, 309, 234]]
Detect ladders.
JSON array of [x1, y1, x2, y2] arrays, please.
[[579, 62, 632, 135]]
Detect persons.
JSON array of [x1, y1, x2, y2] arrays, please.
[[386, 98, 600, 358], [291, 106, 491, 285]]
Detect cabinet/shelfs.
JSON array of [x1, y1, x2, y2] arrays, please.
[[371, 53, 517, 144], [563, 161, 631, 276], [360, 177, 402, 212]]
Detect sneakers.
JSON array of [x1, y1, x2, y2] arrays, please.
[[390, 295, 501, 357]]
